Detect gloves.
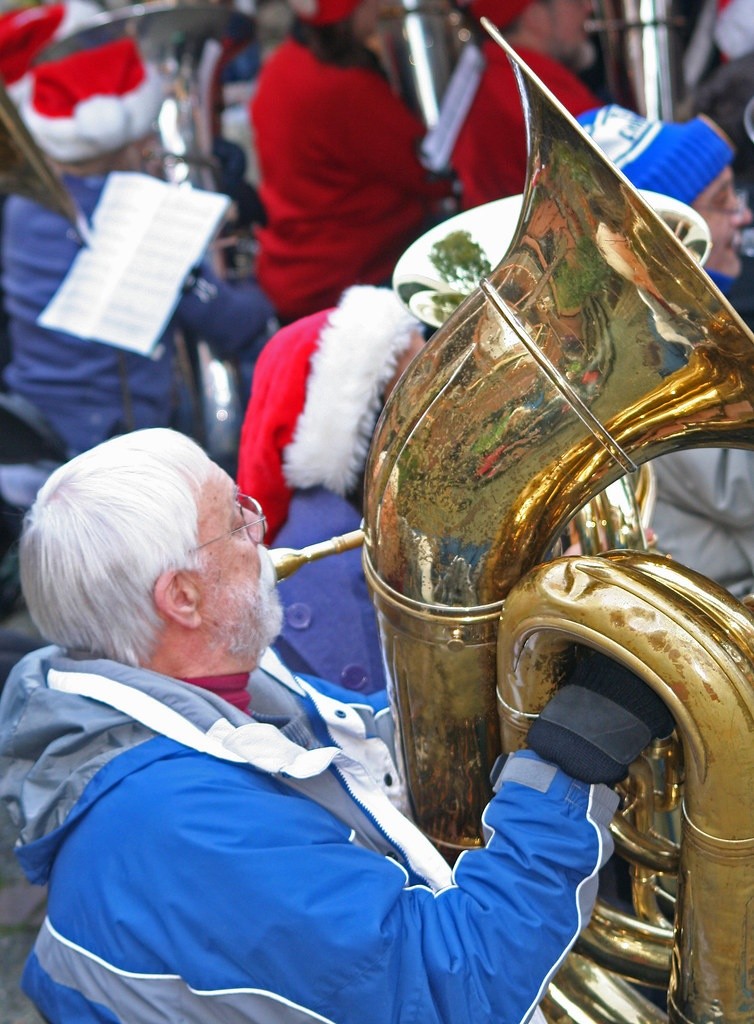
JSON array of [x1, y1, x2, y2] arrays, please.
[[524, 650, 676, 790]]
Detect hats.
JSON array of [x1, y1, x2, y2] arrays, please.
[[288, 0, 361, 25], [712, 0, 754, 66], [5, 37, 157, 163], [0, 2, 112, 104], [575, 103, 735, 206], [236, 285, 425, 549], [469, 0, 534, 30]]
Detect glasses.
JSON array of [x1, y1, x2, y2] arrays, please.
[[188, 493, 266, 554]]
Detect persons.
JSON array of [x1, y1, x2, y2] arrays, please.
[[1, 427, 675, 1024], [0, 0, 753, 779]]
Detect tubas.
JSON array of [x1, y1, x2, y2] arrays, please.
[[362, 0, 681, 222], [235, 11, 754, 1024], [30, 1, 262, 479]]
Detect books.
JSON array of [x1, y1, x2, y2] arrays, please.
[[34, 167, 234, 360]]
